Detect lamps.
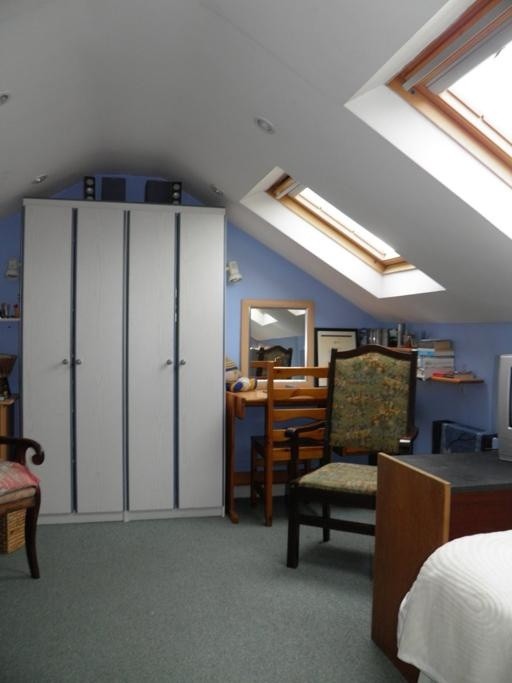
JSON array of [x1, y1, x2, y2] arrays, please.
[[225, 260, 243, 283]]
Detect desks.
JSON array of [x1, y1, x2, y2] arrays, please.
[[372, 449, 511, 679]]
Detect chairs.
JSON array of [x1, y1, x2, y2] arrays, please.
[[250, 344, 418, 569], [0, 435, 43, 578]]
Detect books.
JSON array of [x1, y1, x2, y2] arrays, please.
[[360, 322, 455, 375]]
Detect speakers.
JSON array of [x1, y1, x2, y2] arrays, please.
[[85, 176, 127, 200], [145, 180, 181, 205]]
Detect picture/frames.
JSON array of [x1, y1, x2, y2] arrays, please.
[[314, 328, 360, 389]]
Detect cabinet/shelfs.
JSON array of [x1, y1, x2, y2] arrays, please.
[[22, 198, 226, 525]]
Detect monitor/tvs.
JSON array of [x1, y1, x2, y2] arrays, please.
[[497, 354, 512, 463]]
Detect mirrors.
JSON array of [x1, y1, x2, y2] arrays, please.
[[242, 296, 314, 387]]
